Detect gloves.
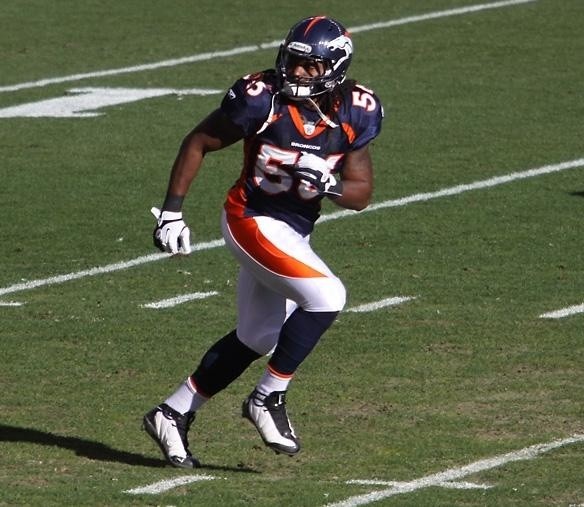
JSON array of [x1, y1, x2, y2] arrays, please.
[[295, 152, 343, 200], [151, 206, 191, 256]]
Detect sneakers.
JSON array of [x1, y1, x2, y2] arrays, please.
[[241, 387, 301, 456], [142, 403, 200, 468]]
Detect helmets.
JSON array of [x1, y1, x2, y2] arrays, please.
[[275, 15, 353, 98]]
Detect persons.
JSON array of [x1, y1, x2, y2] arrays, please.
[[143, 15, 384, 470]]
